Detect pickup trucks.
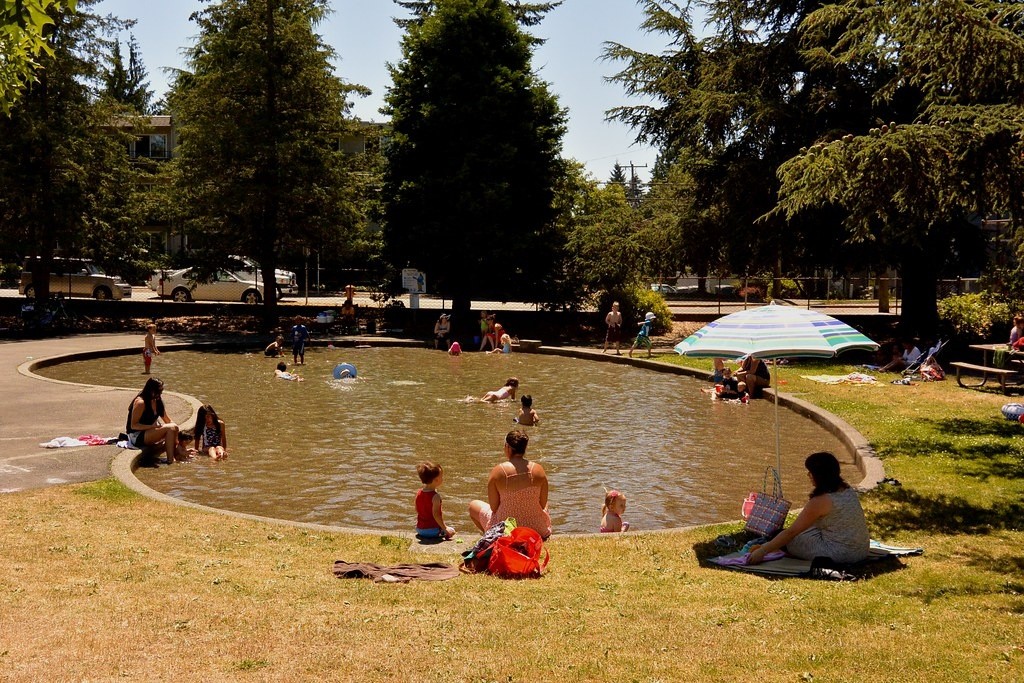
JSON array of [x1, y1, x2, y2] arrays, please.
[[143, 254, 298, 300]]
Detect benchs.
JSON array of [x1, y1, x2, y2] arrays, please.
[[1011, 359, 1024, 364], [950, 361, 1018, 374]]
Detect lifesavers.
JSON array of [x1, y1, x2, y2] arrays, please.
[[333, 363, 358, 379], [1001, 403, 1024, 420]]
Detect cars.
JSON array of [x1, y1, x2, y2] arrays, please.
[[155, 265, 280, 304], [651, 283, 679, 295]]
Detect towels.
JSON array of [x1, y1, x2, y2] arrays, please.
[[716, 550, 785, 566], [332, 559, 460, 583], [39, 437, 117, 448]]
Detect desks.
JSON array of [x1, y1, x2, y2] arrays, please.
[[956, 344, 1024, 393]]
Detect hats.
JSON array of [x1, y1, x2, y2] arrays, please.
[[645, 312, 656, 320], [440, 314, 450, 321]]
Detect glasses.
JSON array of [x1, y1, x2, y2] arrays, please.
[[486, 319, 490, 321]]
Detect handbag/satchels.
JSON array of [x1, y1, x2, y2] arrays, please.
[[458, 517, 549, 576], [743, 466, 792, 538]]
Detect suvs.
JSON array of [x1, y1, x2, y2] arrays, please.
[[17, 255, 132, 300]]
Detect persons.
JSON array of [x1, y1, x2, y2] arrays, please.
[[142, 324, 160, 373], [433, 312, 452, 349], [674, 301, 880, 499], [265, 335, 284, 357], [415, 460, 456, 538], [600, 490, 628, 533], [1009, 316, 1024, 346], [714, 357, 770, 399], [483, 377, 519, 402], [194, 404, 230, 460], [340, 300, 355, 328], [478, 310, 511, 353], [879, 340, 922, 373], [287, 315, 311, 364], [749, 452, 870, 565], [275, 361, 292, 379], [629, 312, 656, 357], [515, 395, 539, 425], [448, 342, 462, 356], [603, 301, 622, 355], [125, 376, 179, 461], [174, 430, 198, 461], [468, 430, 552, 540]]
